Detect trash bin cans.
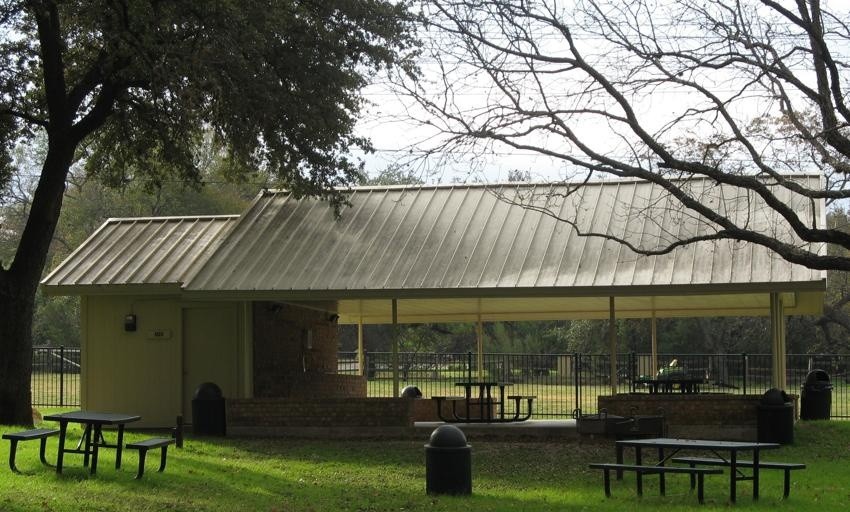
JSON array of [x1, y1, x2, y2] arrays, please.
[[800, 369, 833, 421], [424, 425, 473, 495], [191, 382, 226, 436], [757, 389, 794, 444], [402, 386, 422, 398]]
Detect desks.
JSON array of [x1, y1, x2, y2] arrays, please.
[[44, 411, 141, 476], [456, 382, 513, 423], [634, 379, 703, 393], [616, 438, 780, 503]]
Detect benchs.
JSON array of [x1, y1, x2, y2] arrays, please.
[[432, 396, 464, 423], [126, 438, 175, 478], [589, 464, 723, 505], [508, 396, 536, 422], [672, 457, 806, 499], [2, 429, 60, 475]]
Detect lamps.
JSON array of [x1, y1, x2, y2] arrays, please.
[[267, 304, 282, 313], [328, 314, 339, 322]]
